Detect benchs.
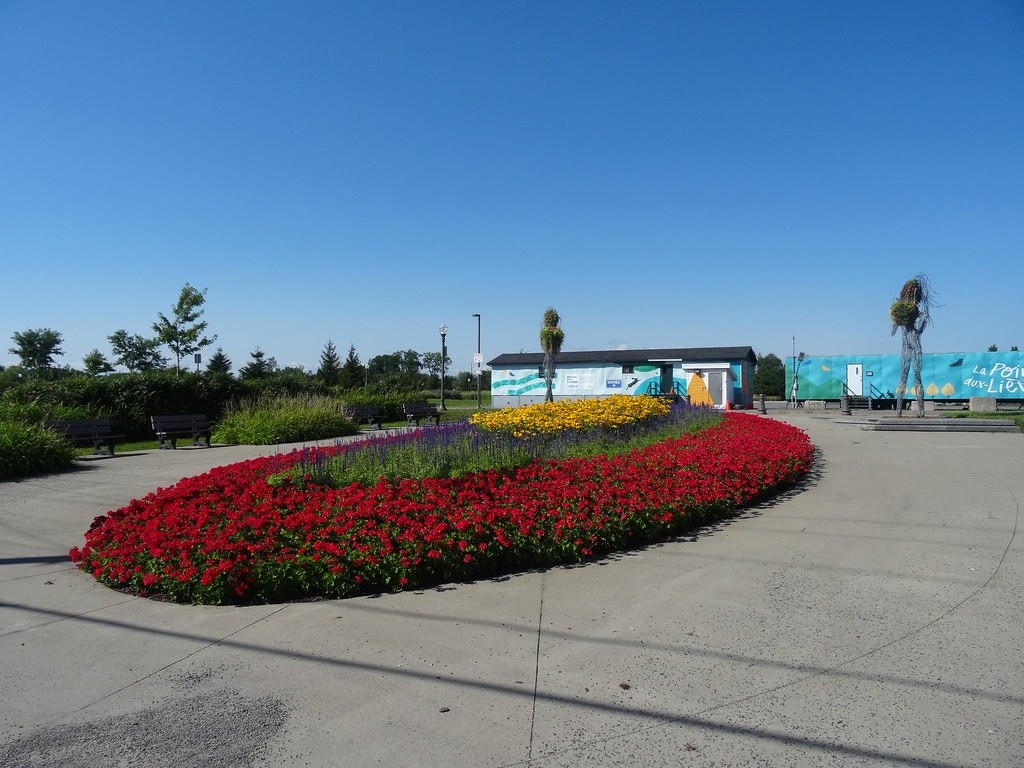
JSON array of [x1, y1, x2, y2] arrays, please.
[[43, 418, 126, 455], [340, 404, 388, 430], [151, 414, 212, 450], [402, 403, 444, 426]]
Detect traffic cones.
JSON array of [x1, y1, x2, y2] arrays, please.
[[669, 386, 676, 394], [726, 398, 732, 410]]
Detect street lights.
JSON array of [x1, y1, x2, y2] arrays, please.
[[438, 322, 449, 412], [472, 313, 486, 409]]
